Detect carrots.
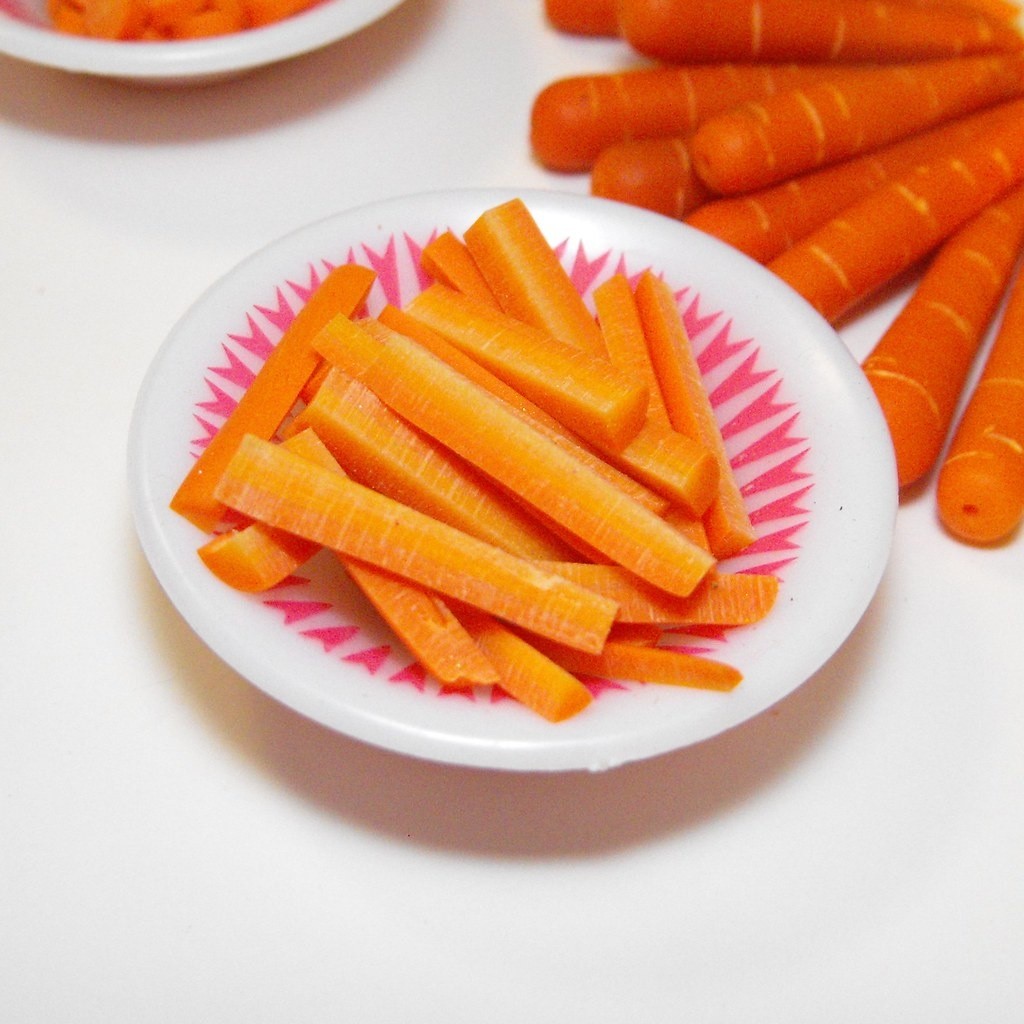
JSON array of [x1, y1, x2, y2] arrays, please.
[[170, 199, 783, 720], [529, 0, 1023, 544], [43, 0, 330, 41]]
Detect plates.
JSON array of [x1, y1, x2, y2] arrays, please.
[[1, 0, 404, 82], [124, 190, 899, 770]]
